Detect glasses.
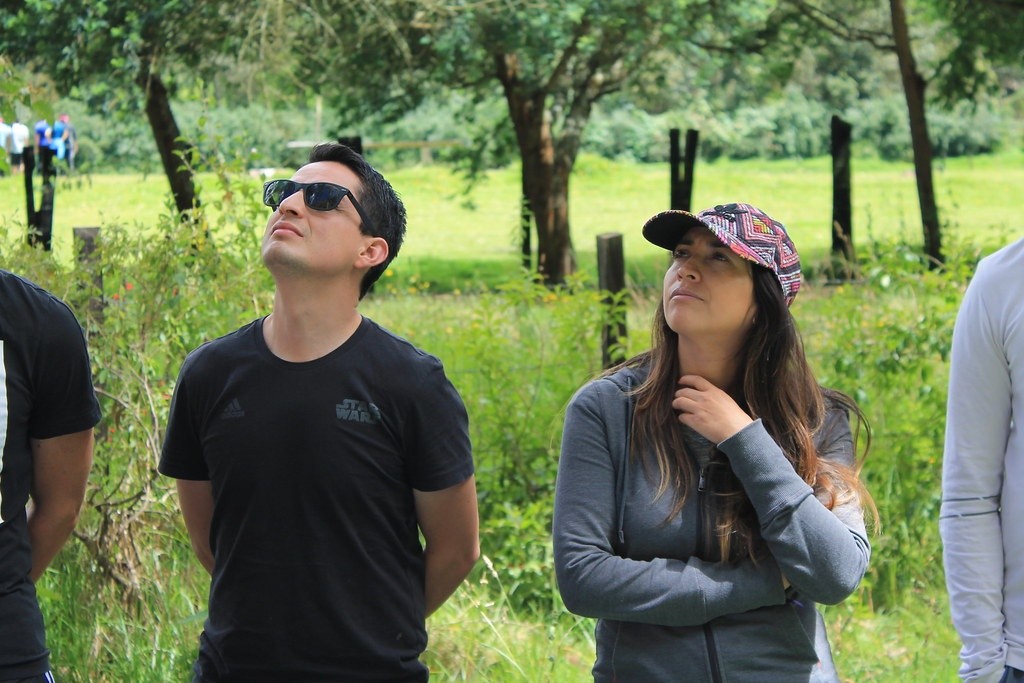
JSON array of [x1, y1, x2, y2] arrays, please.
[[263, 179, 378, 238]]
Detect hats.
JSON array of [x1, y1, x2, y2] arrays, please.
[[641, 202, 802, 308]]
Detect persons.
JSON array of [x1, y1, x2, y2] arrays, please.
[[157, 143, 479, 683], [0, 267, 103, 683], [0, 115, 78, 176], [552, 205, 882, 683], [939, 238, 1024, 683]]
